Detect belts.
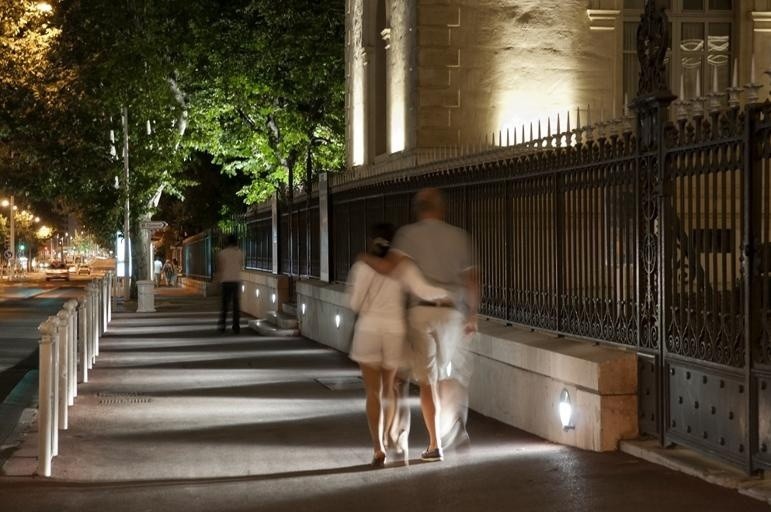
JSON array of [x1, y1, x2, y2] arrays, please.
[[417, 300, 456, 309]]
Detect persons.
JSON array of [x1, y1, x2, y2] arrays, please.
[[154, 257, 163, 287], [172, 258, 179, 286], [345, 221, 446, 468], [215, 235, 246, 335], [161, 260, 174, 287], [357, 186, 486, 464]]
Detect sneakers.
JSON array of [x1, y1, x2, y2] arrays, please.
[[420, 447, 443, 461], [216, 324, 241, 336]]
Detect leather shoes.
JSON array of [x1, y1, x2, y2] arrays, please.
[[371, 450, 386, 468]]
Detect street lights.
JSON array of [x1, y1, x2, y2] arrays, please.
[[28, 217, 40, 271], [1, 196, 15, 276]]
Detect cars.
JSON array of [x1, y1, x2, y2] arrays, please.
[[46, 256, 90, 281]]
